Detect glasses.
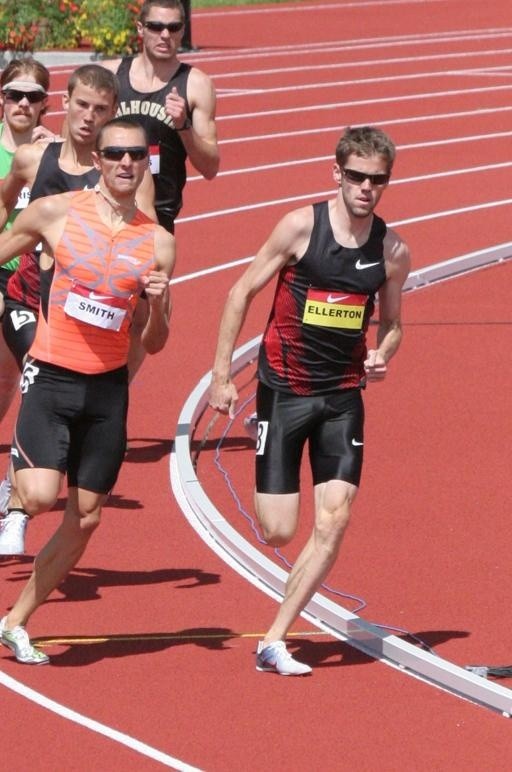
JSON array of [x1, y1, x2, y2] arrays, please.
[[145, 22, 184, 32], [1, 90, 47, 103], [342, 168, 389, 185], [97, 148, 148, 161]]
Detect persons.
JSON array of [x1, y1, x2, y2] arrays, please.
[[96, 1, 217, 230], [2, 115, 183, 668], [207, 125, 412, 679], [2, 62, 163, 560], [0, 57, 61, 296]]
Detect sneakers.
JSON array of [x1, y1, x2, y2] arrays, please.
[[0, 464, 13, 516], [255, 640, 312, 676], [0, 511, 30, 555], [0, 614, 50, 665], [243, 411, 257, 440]]
